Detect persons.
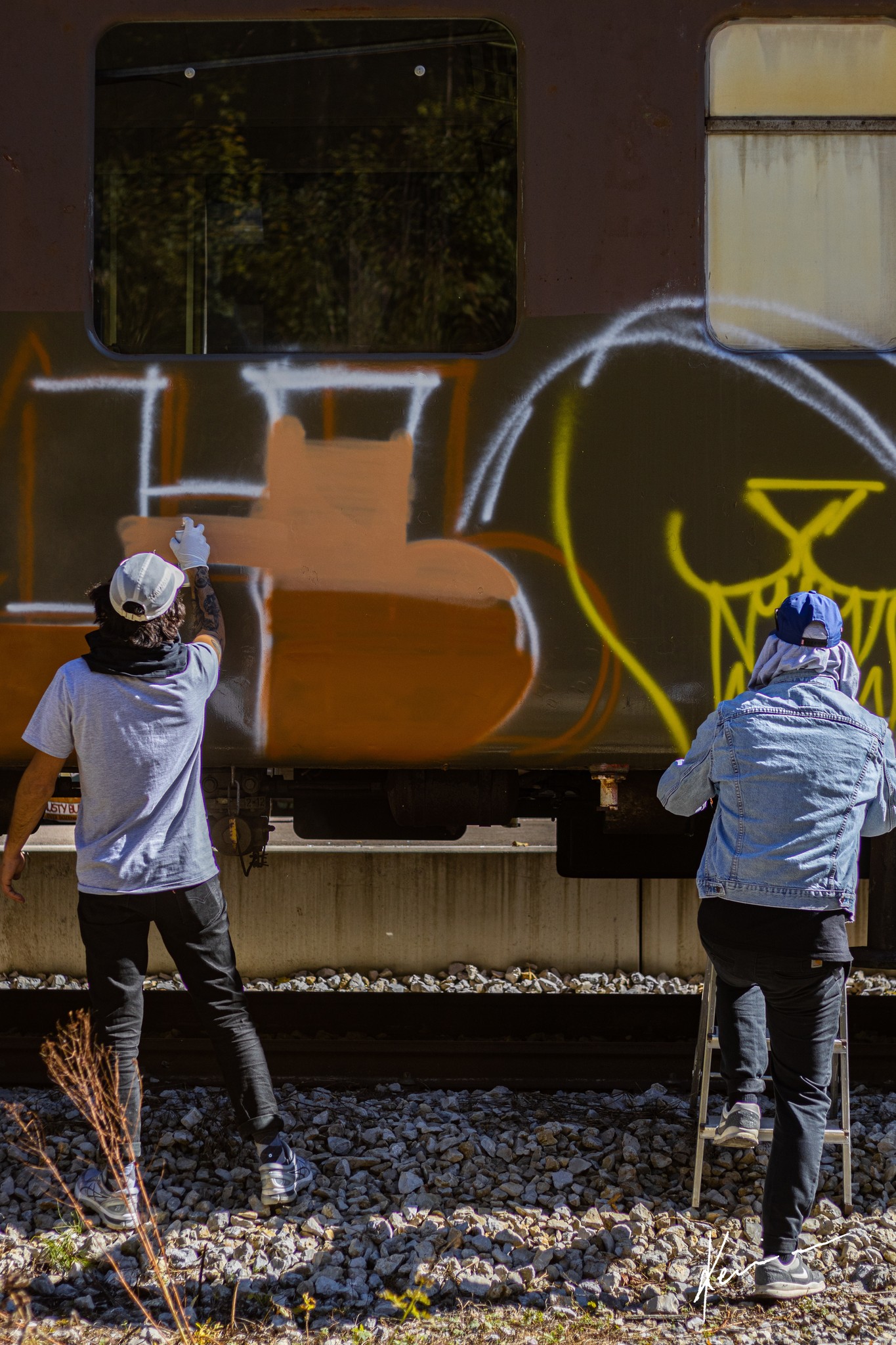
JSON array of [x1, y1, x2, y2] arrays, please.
[[0, 515, 320, 1232], [656, 588, 896, 1298]]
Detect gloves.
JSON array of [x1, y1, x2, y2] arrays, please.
[[169, 517, 210, 576]]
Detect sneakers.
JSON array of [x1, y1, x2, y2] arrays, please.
[[75, 1168, 141, 1231], [258, 1134, 313, 1205], [713, 1101, 761, 1148], [752, 1253, 826, 1299]]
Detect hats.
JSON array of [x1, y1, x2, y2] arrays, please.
[[768, 590, 843, 647], [109, 552, 186, 622]]
